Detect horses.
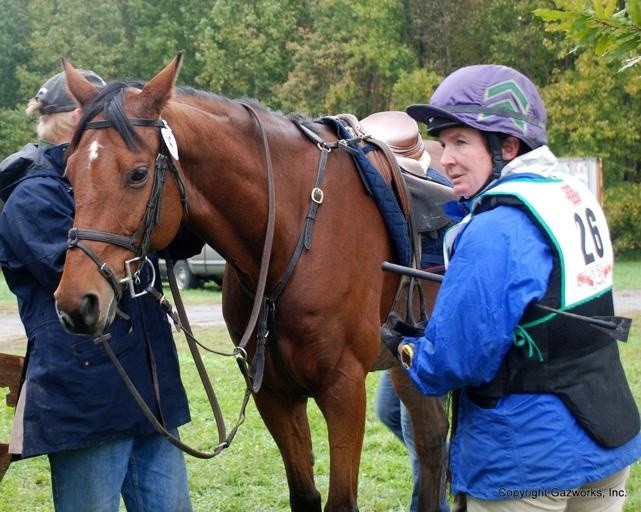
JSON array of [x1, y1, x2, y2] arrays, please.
[[54, 52, 461, 511]]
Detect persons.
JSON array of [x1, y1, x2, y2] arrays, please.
[[374, 369, 453, 511], [378, 62, 640, 511], [0, 69, 193, 511]]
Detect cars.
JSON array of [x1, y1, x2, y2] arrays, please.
[[158, 243, 227, 291]]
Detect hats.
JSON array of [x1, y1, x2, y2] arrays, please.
[[34, 69, 107, 114]]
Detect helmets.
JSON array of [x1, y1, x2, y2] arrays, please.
[[407, 64, 548, 150]]
